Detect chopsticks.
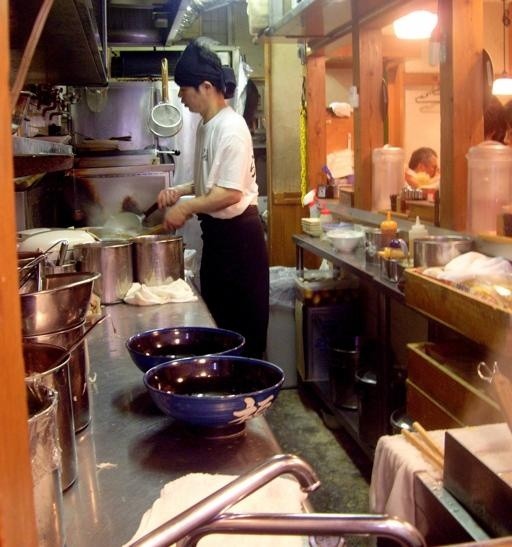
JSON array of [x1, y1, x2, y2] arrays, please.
[[404, 418, 445, 473]]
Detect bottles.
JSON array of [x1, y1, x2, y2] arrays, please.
[[379, 210, 398, 231], [310, 202, 332, 233], [408, 216, 427, 248]]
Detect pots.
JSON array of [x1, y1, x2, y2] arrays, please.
[[313, 330, 408, 445], [42, 235, 191, 301], [22, 313, 111, 547], [413, 236, 475, 267]]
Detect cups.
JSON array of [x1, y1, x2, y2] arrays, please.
[[366, 229, 396, 264]]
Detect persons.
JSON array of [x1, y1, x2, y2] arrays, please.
[[482, 94, 512, 145], [400, 146, 439, 189], [157, 38, 270, 365]]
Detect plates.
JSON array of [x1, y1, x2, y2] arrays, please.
[[300, 214, 320, 237]]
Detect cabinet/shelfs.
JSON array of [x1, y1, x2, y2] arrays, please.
[[0, 2, 115, 164], [395, 267, 512, 449]]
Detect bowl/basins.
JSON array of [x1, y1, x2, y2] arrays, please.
[[126, 325, 284, 439], [79, 140, 121, 153], [21, 272, 105, 336], [328, 230, 365, 254]]
